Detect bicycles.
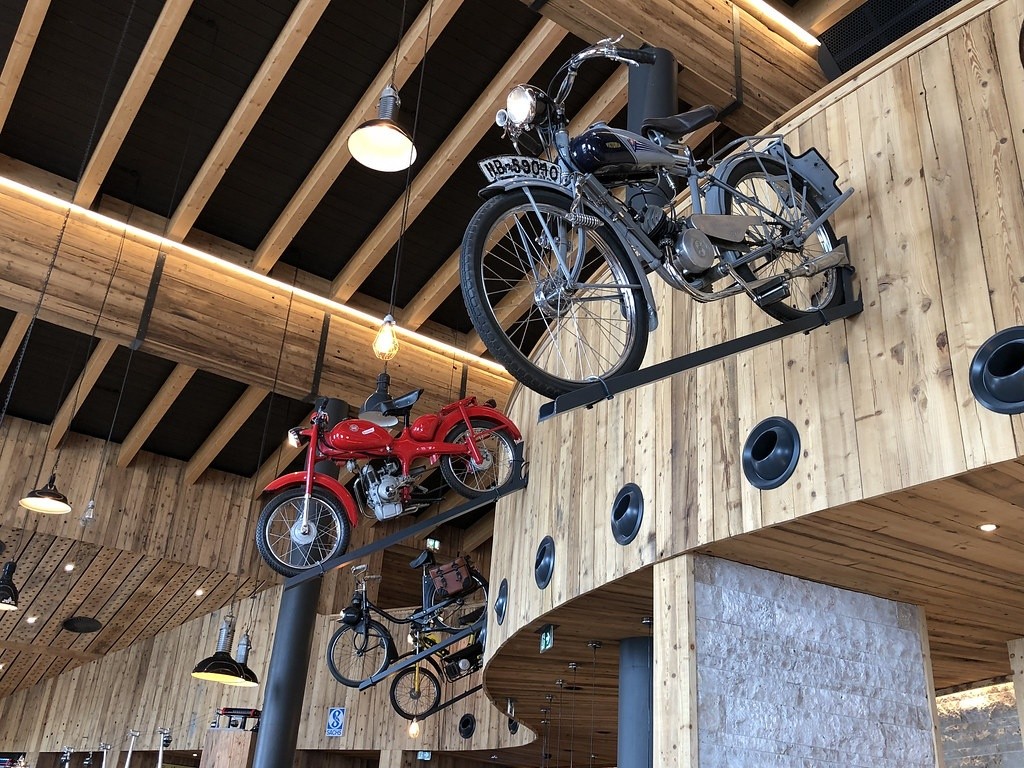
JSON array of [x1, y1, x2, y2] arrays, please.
[[327, 549, 488, 688]]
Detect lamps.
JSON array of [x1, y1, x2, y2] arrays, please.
[[0, 5, 301, 689], [348, 0, 418, 173]]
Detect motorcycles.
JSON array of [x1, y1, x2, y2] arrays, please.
[[389, 619, 486, 721], [256, 386, 522, 578], [458, 34, 854, 399]]
